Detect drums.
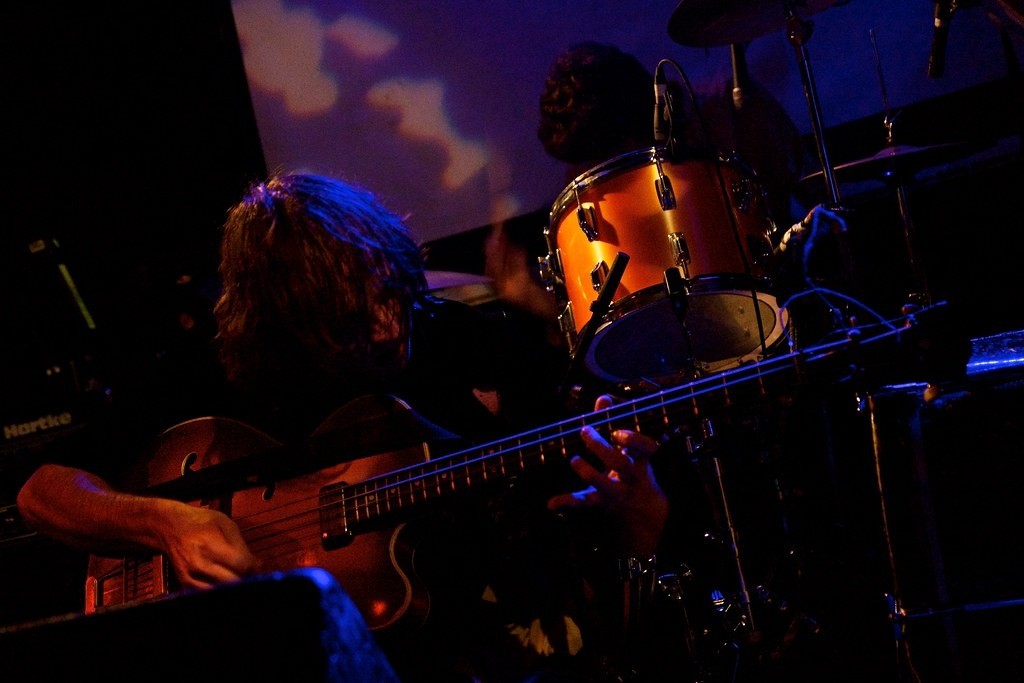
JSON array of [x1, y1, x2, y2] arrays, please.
[[544, 142, 1024, 621], [431, 285, 503, 325]]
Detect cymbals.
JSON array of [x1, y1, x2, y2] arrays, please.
[[425, 270, 492, 290], [667, 0, 839, 49], [799, 142, 996, 183]]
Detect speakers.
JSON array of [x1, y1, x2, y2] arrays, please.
[[0, 566, 400, 683]]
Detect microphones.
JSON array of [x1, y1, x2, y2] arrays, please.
[[928, 0, 956, 79], [730, 42, 753, 111], [653, 65, 670, 141]]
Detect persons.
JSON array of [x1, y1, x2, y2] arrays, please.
[[16, 171, 669, 683], [487, 40, 799, 358]]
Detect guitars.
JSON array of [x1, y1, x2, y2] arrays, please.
[[85, 291, 972, 642]]
